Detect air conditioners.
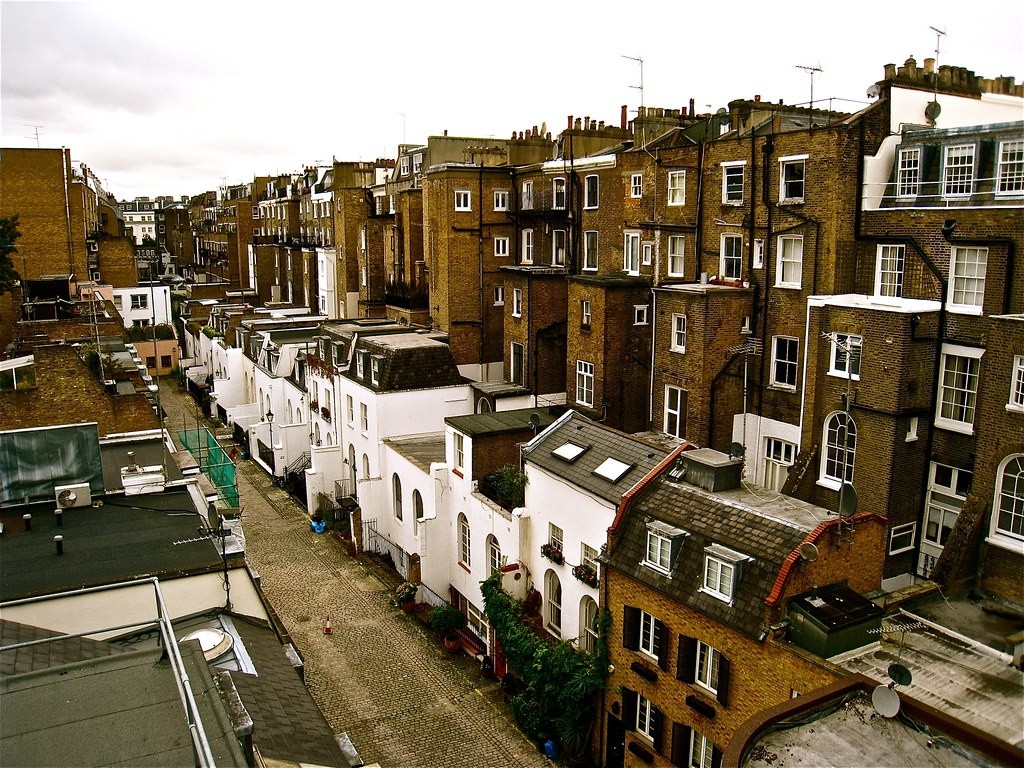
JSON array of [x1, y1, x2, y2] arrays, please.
[[54, 483, 92, 509]]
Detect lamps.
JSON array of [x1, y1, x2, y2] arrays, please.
[[317, 440, 322, 446], [309, 433, 314, 439], [215, 370, 219, 376], [204, 361, 207, 366], [344, 458, 349, 464]]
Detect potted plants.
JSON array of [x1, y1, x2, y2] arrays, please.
[[346, 542, 356, 557], [334, 519, 351, 539], [326, 521, 334, 529], [429, 603, 467, 650]]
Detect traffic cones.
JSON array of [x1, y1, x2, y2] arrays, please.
[[323, 614, 333, 634]]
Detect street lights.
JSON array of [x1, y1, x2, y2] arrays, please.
[[266, 408, 275, 483]]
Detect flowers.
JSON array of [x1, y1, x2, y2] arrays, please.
[[396, 581, 418, 602], [541, 544, 561, 564], [574, 565, 597, 583]]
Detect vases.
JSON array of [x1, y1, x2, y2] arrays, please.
[[543, 550, 562, 565], [576, 574, 597, 589], [401, 599, 416, 614]]
